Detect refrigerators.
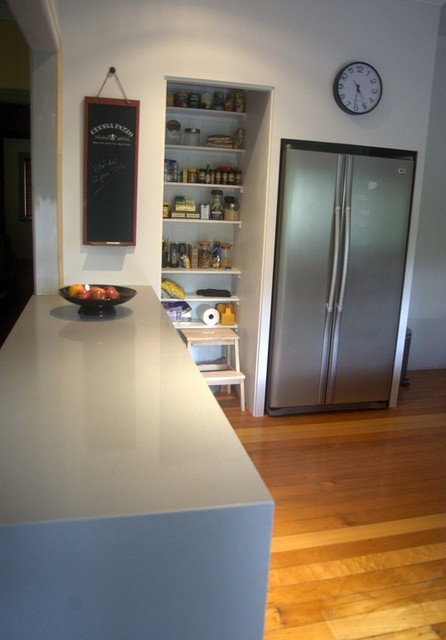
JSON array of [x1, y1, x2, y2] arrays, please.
[[265, 139, 418, 416]]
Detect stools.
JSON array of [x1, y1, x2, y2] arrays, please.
[[179, 328, 246, 412]]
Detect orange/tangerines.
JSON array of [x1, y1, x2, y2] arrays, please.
[[69, 284, 84, 297]]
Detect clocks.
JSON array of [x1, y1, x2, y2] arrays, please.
[[333, 61, 383, 115]]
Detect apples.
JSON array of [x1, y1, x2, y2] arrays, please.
[[91, 290, 105, 301], [105, 287, 116, 290], [79, 290, 92, 300], [106, 291, 119, 300]]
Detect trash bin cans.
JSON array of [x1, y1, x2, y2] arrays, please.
[[399, 328, 411, 385]]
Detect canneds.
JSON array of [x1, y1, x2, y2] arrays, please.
[[174, 90, 188, 108], [199, 169, 206, 182], [187, 168, 197, 182], [189, 93, 200, 108]]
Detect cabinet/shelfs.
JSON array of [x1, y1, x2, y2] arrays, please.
[[160, 83, 249, 329]]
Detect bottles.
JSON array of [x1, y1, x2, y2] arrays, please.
[[163, 202, 169, 218], [210, 190, 224, 220], [220, 243, 232, 269], [198, 241, 212, 269], [187, 164, 241, 184]]
